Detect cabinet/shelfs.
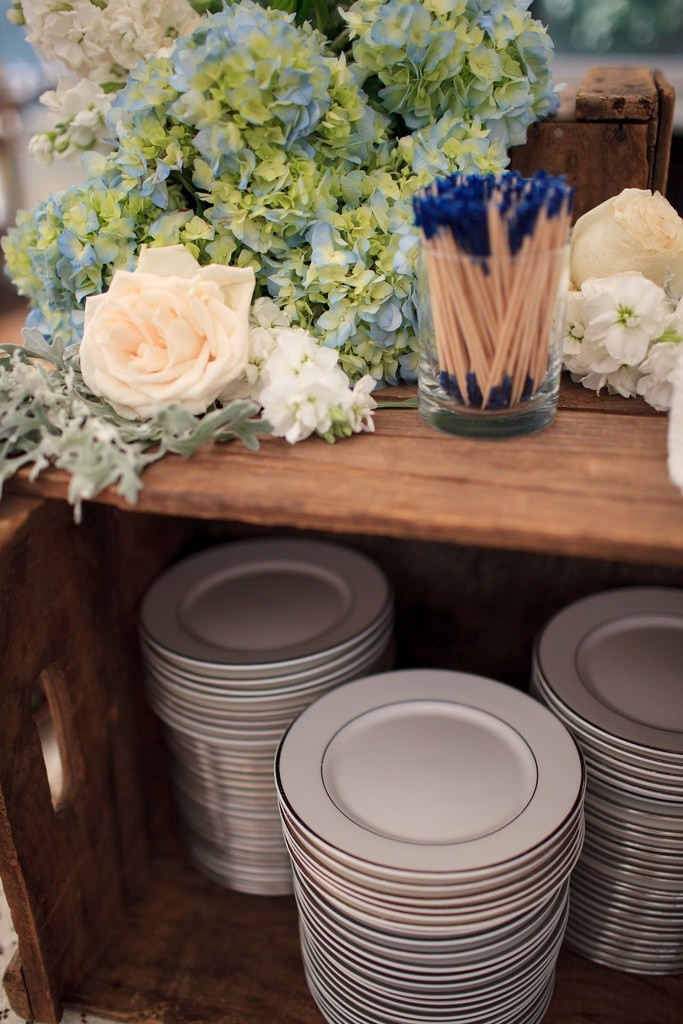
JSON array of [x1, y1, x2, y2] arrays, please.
[[1, 377, 681, 1023]]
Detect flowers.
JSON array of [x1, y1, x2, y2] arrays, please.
[[0, 0, 683, 512]]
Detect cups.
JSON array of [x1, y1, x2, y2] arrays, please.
[[416, 232, 574, 442]]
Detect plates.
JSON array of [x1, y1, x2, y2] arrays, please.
[[275, 669, 587, 1024], [532, 586, 683, 975], [139, 538, 393, 898]]
